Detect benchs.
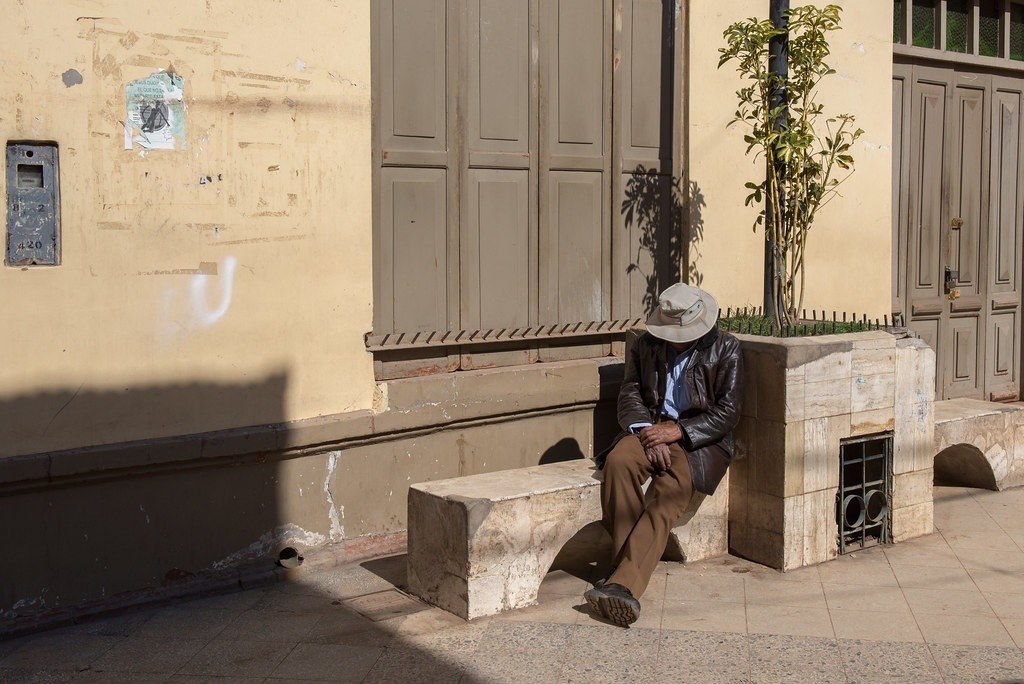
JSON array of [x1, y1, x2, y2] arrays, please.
[[405, 458, 729, 620], [932, 398, 1024, 492]]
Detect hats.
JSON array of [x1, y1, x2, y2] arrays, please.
[[645, 283, 720, 343]]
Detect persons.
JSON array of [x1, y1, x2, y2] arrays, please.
[[586, 282, 744, 625]]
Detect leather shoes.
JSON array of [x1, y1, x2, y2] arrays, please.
[[582, 584, 641, 625], [595, 567, 617, 589]]
